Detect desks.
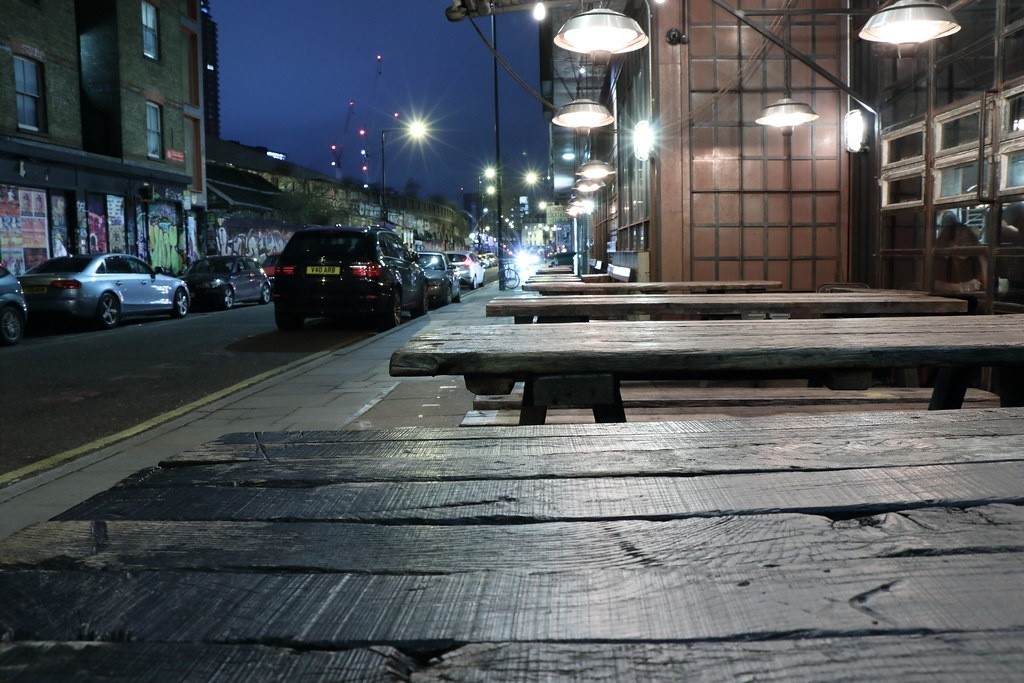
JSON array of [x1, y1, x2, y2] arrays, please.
[[0, 264, 1024, 683]]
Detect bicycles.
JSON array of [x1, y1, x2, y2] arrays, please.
[[498, 259, 520, 289]]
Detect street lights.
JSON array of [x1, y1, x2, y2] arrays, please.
[[381, 123, 426, 219], [478, 167, 496, 250], [526, 170, 548, 223]]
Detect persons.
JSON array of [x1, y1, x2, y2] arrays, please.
[[932, 224, 989, 293]]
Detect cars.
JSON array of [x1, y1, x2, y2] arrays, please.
[[416, 250, 462, 308], [262, 251, 282, 285], [15, 253, 193, 329], [0, 262, 29, 347], [439, 250, 486, 290], [173, 255, 273, 311], [478, 252, 519, 269]]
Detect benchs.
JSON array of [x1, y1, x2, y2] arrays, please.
[[473, 388, 1001, 407], [459, 402, 930, 426]]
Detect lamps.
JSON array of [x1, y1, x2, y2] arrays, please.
[[553, 0, 963, 214]]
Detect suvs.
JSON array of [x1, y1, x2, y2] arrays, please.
[[272, 222, 429, 332]]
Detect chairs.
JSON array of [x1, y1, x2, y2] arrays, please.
[[817, 283, 870, 292]]
